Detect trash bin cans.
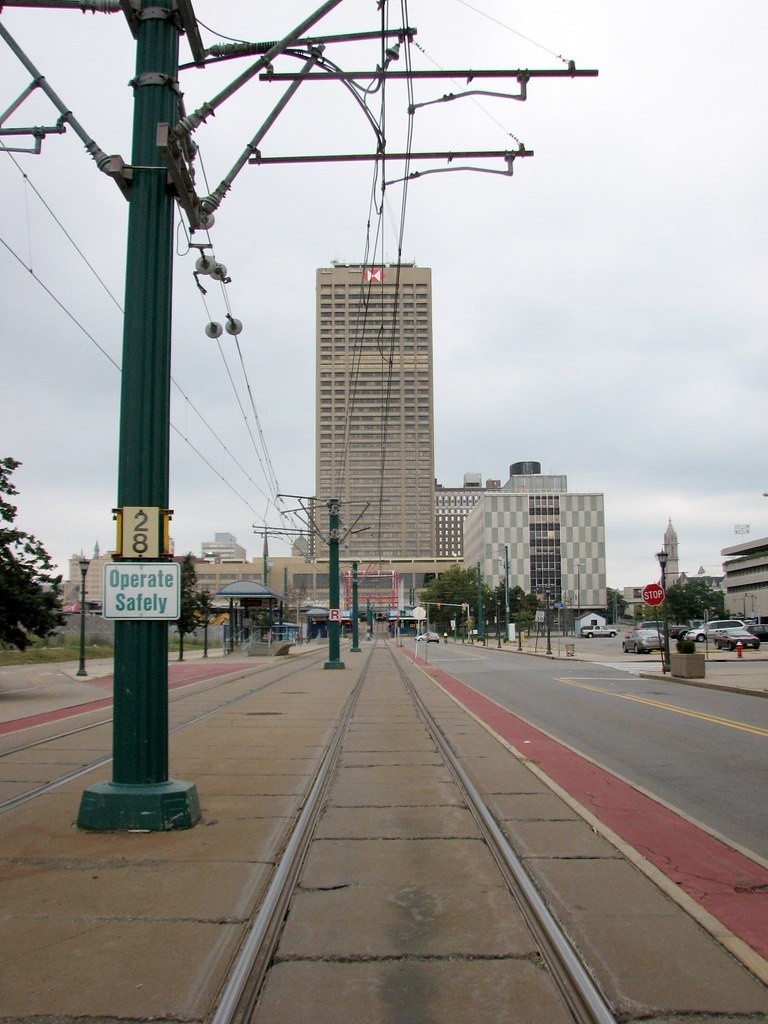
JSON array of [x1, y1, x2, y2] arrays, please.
[[566, 644, 574, 656]]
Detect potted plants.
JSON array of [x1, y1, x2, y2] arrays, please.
[[670, 638, 705, 679]]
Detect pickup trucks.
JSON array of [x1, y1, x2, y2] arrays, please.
[[658, 619, 710, 641], [581, 625, 618, 638]]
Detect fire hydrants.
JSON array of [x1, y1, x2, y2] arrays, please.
[[521, 631, 525, 643], [735, 640, 743, 658]]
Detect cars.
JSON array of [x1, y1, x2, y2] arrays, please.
[[747, 624, 768, 641], [714, 629, 761, 651], [621, 630, 665, 654], [683, 620, 747, 643], [427, 633, 439, 643], [414, 632, 430, 641]]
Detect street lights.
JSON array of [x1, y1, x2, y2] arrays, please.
[[76, 557, 90, 676], [733, 598, 745, 620], [577, 563, 585, 617], [517, 594, 524, 651], [481, 604, 488, 646], [496, 600, 502, 648], [656, 547, 673, 673], [545, 587, 554, 655]]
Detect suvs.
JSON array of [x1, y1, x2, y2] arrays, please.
[[633, 621, 673, 632]]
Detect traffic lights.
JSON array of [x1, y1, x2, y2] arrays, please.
[[465, 619, 475, 629]]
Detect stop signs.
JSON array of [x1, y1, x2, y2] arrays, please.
[[642, 583, 665, 607]]
